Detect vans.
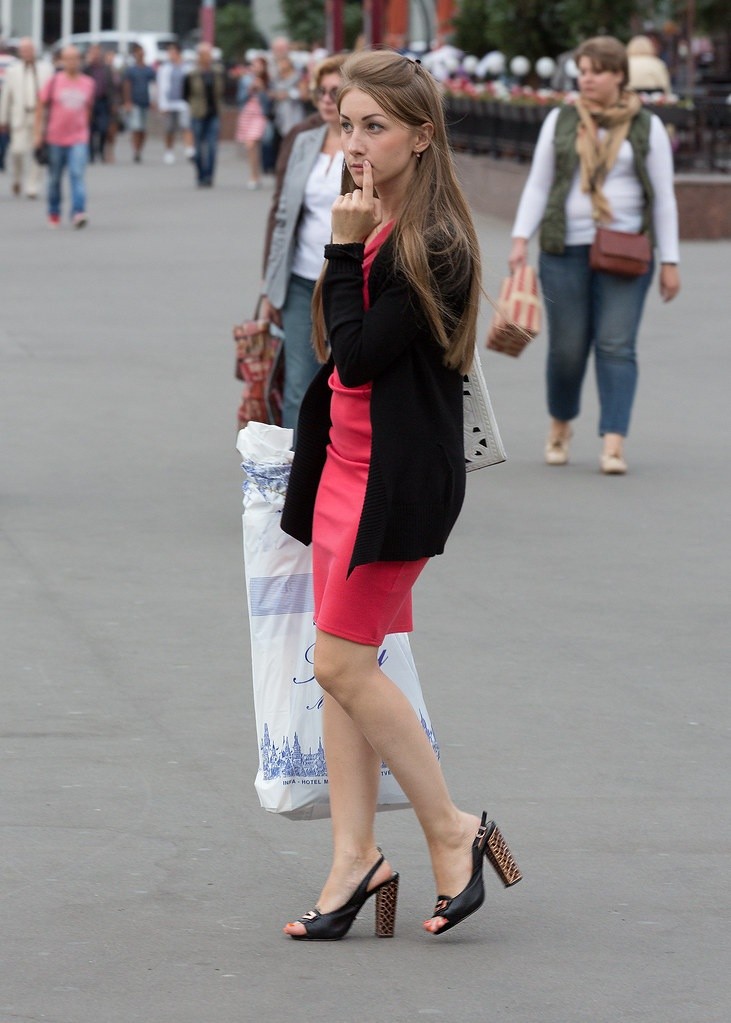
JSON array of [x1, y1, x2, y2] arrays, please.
[[42, 32, 180, 71]]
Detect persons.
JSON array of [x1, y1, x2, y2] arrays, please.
[[255, 57, 342, 449], [158, 42, 196, 165], [183, 41, 232, 188], [238, 49, 333, 190], [124, 43, 158, 164], [31, 44, 96, 230], [506, 36, 681, 475], [104, 48, 123, 164], [82, 43, 117, 163], [280, 51, 521, 939], [0, 35, 54, 200]]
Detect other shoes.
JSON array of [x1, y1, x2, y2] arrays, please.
[[185, 148, 194, 157], [250, 182, 260, 189], [164, 152, 174, 162], [49, 213, 61, 228], [601, 452, 628, 474], [70, 213, 89, 232], [544, 436, 571, 465]]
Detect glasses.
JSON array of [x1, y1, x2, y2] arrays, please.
[[311, 86, 340, 105]]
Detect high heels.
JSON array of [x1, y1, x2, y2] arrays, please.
[[432, 809, 525, 936], [291, 847, 400, 941]]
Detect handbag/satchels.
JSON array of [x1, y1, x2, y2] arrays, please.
[[34, 143, 50, 164], [232, 319, 285, 432], [589, 227, 653, 280], [485, 264, 543, 361], [237, 415, 441, 821], [459, 341, 510, 474]]
